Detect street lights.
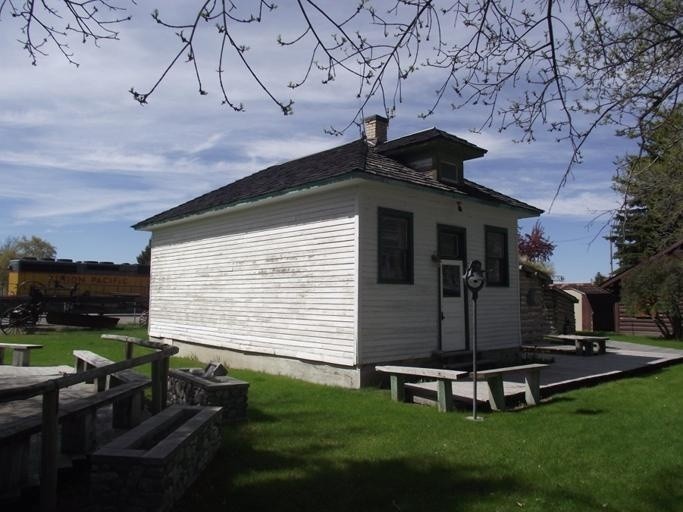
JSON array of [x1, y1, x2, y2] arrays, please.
[[463, 259, 486, 420]]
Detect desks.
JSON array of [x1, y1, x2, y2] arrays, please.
[[545, 334, 610, 355]]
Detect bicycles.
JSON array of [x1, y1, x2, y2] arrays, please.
[[0, 275, 80, 334]]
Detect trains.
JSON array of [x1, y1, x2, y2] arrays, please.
[[7, 257, 149, 313]]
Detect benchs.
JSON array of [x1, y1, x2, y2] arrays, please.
[[375, 365, 469, 413], [469, 364, 550, 411], [0, 342, 153, 490]]
